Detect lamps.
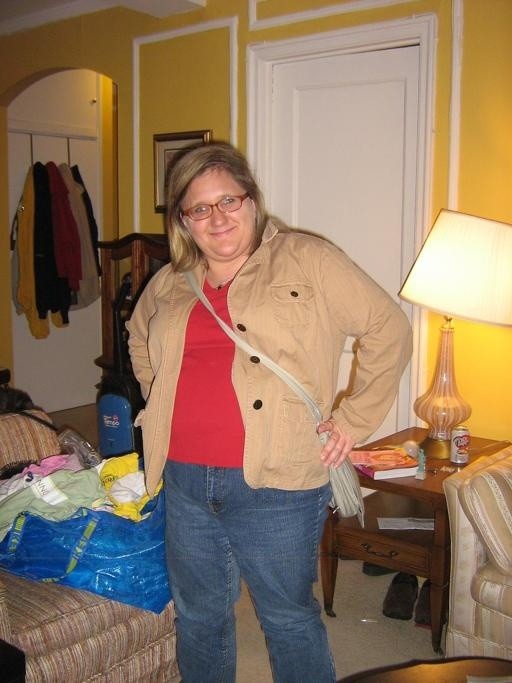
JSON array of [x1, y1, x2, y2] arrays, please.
[[398, 209, 512, 460]]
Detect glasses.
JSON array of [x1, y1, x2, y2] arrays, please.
[[184, 191, 249, 221]]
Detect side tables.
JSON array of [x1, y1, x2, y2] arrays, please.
[[321, 426, 512, 652]]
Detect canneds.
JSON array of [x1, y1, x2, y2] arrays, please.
[[449, 426, 470, 464]]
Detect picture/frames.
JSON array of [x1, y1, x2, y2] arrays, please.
[[153, 130, 210, 213]]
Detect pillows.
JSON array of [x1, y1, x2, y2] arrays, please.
[[459, 456, 512, 575]]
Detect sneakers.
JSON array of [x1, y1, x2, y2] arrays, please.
[[381, 571, 431, 627]]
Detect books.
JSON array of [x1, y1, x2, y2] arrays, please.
[[348, 446, 420, 480]]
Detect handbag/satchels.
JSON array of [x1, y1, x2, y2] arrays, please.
[[1, 488, 172, 614]]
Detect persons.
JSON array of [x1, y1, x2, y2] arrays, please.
[[125, 142, 413, 682]]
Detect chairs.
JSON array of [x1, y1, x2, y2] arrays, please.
[[441, 446, 511, 661], [0, 409, 182, 683]]
